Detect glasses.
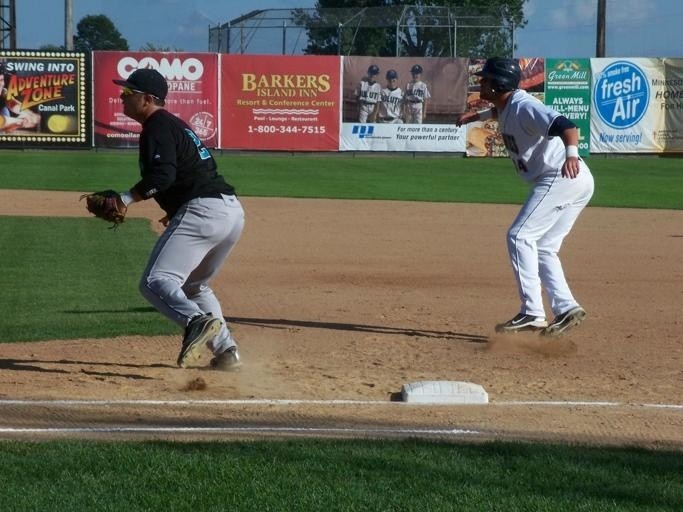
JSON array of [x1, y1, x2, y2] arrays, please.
[[124, 87, 159, 101]]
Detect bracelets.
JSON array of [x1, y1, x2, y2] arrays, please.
[[118, 191, 135, 207], [565, 144, 579, 160], [476, 108, 492, 120]]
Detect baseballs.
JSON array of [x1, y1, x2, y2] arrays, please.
[[48, 115, 68, 132]]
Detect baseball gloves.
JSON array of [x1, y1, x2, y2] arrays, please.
[[86, 189, 127, 223]]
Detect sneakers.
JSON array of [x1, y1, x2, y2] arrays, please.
[[177, 313, 221, 369], [210, 346, 242, 369], [495, 307, 586, 336]]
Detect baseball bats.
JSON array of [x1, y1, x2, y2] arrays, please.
[[0, 115, 24, 129]]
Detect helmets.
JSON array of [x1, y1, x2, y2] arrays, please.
[[472, 57, 520, 92]]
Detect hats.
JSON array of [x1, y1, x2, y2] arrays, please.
[[368, 66, 379, 74], [386, 70, 397, 78], [113, 70, 168, 100], [409, 65, 422, 73]]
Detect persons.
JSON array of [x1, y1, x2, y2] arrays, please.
[[0, 64, 41, 135], [352, 65, 382, 122], [403, 65, 431, 124], [377, 70, 404, 124], [456, 58, 594, 340], [82, 66, 246, 375]]
